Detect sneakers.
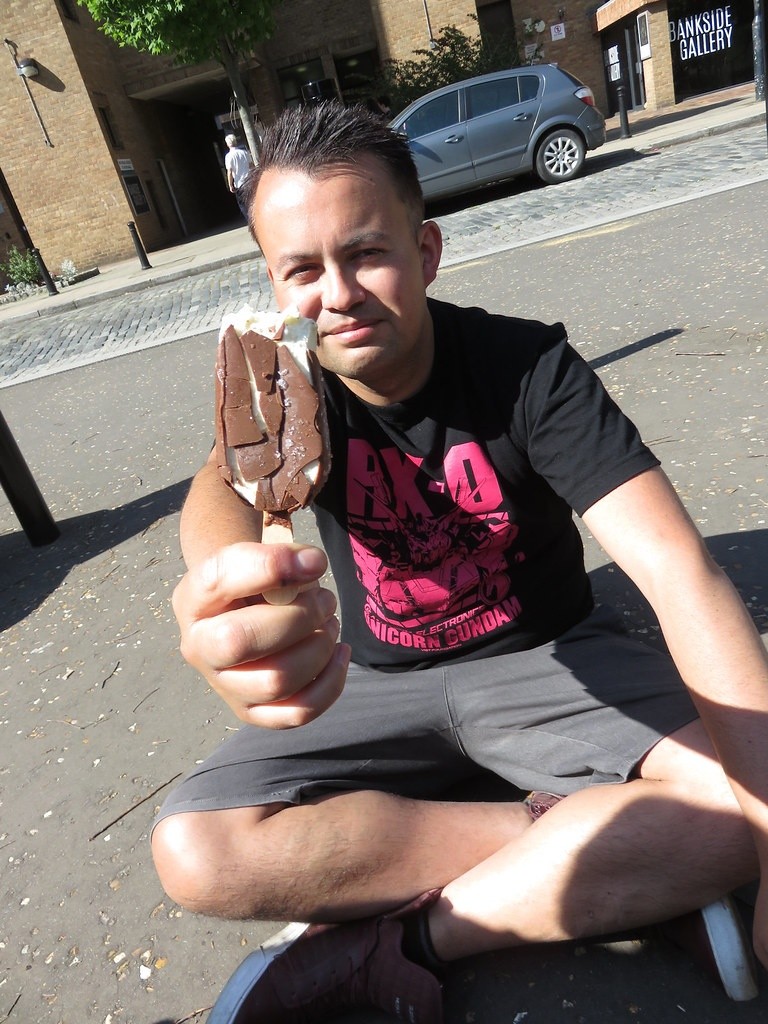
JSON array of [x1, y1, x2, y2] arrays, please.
[[524, 789, 758, 1001], [205, 886, 444, 1024]]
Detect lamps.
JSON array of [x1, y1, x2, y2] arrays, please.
[[19, 58, 39, 79]]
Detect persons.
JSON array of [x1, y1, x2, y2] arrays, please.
[[153, 97, 768, 1024], [225, 134, 255, 224], [377, 95, 397, 123]]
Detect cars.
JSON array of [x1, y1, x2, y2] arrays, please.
[[385, 61, 606, 204]]
